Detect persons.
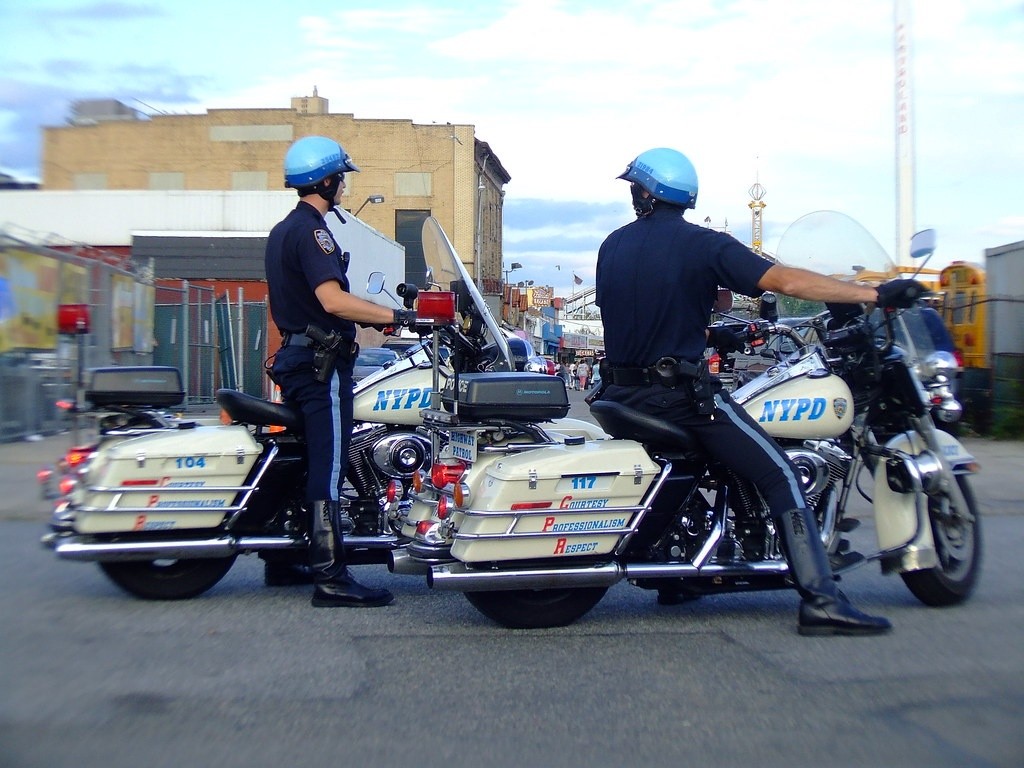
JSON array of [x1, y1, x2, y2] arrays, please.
[[555, 361, 600, 391], [593, 148, 932, 636], [263, 137, 432, 609]]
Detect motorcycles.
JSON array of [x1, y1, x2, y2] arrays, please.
[[37, 217, 613, 601], [387, 228, 985, 629]]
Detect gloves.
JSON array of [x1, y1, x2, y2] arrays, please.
[[873, 280, 927, 309], [706, 326, 745, 355], [392, 311, 433, 336]]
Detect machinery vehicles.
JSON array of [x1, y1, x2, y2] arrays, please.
[[826, 261, 987, 432]]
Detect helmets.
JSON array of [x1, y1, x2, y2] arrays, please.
[[283, 136, 360, 189], [615, 148, 698, 209]]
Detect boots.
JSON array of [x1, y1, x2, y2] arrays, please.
[[657, 589, 702, 606], [304, 500, 395, 608], [773, 508, 893, 637], [265, 563, 324, 587]]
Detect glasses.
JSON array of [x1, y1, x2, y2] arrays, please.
[[339, 174, 344, 182]]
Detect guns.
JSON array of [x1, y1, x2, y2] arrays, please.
[[305, 323, 344, 382], [675, 358, 713, 419]]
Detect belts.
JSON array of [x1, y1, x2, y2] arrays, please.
[[598, 364, 652, 388], [288, 333, 360, 358]]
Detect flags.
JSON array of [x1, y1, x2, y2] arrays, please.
[[574, 274, 583, 285]]
